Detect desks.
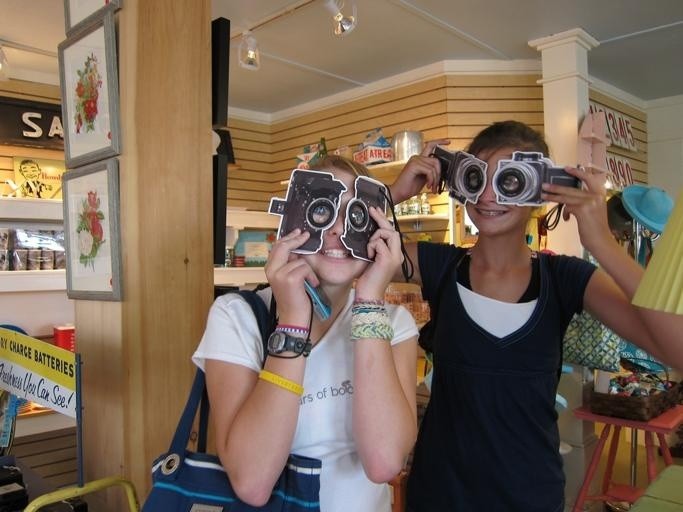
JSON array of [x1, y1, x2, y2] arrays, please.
[[572, 400, 683, 512]]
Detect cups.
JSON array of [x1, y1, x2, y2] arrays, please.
[[1, 226, 66, 270]]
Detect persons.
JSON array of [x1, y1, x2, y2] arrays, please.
[[189, 156, 420, 511], [386, 120, 683, 512]]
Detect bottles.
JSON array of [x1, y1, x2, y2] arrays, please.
[[394, 194, 430, 214]]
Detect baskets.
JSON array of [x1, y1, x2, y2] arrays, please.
[[584, 359, 683, 422]]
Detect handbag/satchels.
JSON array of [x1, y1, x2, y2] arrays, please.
[[138, 446, 322, 512], [563, 306, 676, 373]]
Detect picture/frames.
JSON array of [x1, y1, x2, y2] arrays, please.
[[54, 0, 124, 303]]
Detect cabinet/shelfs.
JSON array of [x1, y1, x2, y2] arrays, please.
[[1, 196, 284, 295], [277, 157, 450, 224]]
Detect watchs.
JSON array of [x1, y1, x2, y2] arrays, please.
[[263, 333, 311, 356]]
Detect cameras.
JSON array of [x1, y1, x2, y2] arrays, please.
[[268, 167, 346, 258], [305, 276, 332, 320], [490, 150, 581, 207], [340, 173, 389, 264], [429, 142, 488, 207]]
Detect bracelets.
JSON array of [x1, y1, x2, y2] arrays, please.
[[349, 298, 394, 339], [272, 323, 310, 338], [258, 369, 304, 396]]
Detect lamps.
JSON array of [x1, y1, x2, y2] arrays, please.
[[238, 0, 357, 73]]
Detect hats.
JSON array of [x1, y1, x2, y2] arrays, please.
[[619, 183, 674, 235]]
